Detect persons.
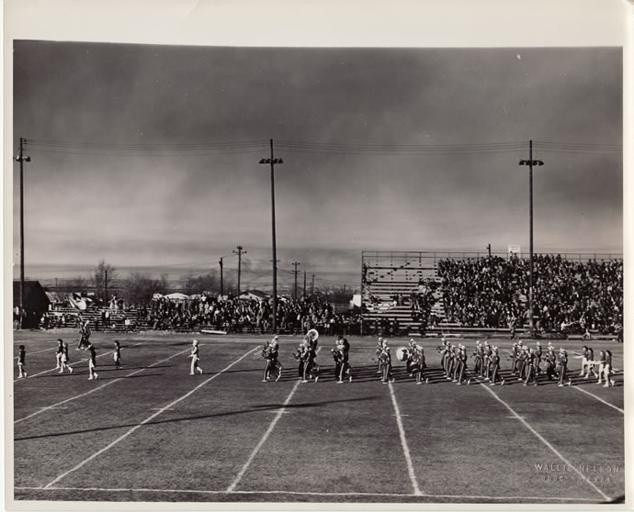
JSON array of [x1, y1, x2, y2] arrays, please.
[[54, 338, 63, 370], [59, 342, 74, 375], [17, 344, 29, 379], [260, 334, 619, 386], [13, 245, 624, 346], [187, 340, 204, 377], [82, 342, 102, 382], [111, 339, 126, 371]]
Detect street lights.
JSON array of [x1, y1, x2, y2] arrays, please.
[[518, 140, 548, 337], [258, 137, 288, 334], [217, 246, 317, 304], [14, 139, 33, 333]]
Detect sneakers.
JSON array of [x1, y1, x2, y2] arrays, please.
[[301, 364, 320, 385], [376, 370, 395, 386], [336, 365, 353, 384], [189, 368, 204, 376], [261, 364, 283, 384], [75, 343, 93, 352], [579, 368, 620, 388], [87, 372, 99, 381], [510, 370, 573, 388], [17, 371, 29, 379], [442, 368, 471, 386], [56, 365, 74, 374], [478, 371, 506, 386], [409, 373, 429, 385]]
